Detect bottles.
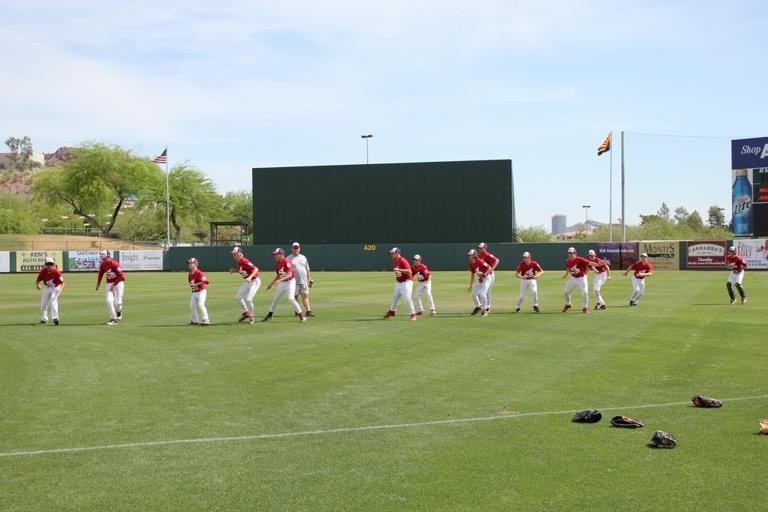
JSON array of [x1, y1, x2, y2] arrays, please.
[[732, 169, 754, 236]]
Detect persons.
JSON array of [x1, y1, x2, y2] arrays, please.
[[261, 248, 307, 321], [466, 249, 491, 318], [515, 251, 543, 312], [477, 243, 500, 313], [96, 250, 125, 325], [622, 253, 652, 306], [229, 247, 261, 325], [383, 247, 417, 321], [285, 242, 316, 317], [585, 250, 612, 309], [725, 246, 748, 305], [561, 247, 607, 313], [410, 254, 436, 315], [35, 257, 64, 325], [186, 257, 210, 325]]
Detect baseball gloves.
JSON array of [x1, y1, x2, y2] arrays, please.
[[646, 430, 676, 450], [610, 415, 643, 430], [570, 410, 603, 425], [690, 393, 722, 410]]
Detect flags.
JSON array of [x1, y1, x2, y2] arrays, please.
[[597, 133, 612, 157], [152, 148, 167, 163]]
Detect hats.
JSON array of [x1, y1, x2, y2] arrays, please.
[[45, 257, 55, 265], [99, 250, 110, 259], [187, 242, 300, 265], [388, 242, 648, 258], [728, 246, 736, 252]]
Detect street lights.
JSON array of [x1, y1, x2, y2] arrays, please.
[[581, 205, 591, 233], [360, 134, 372, 164]]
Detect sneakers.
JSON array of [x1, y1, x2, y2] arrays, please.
[[384, 300, 638, 321], [41, 311, 316, 325], [729, 298, 736, 305], [742, 297, 747, 304]]
